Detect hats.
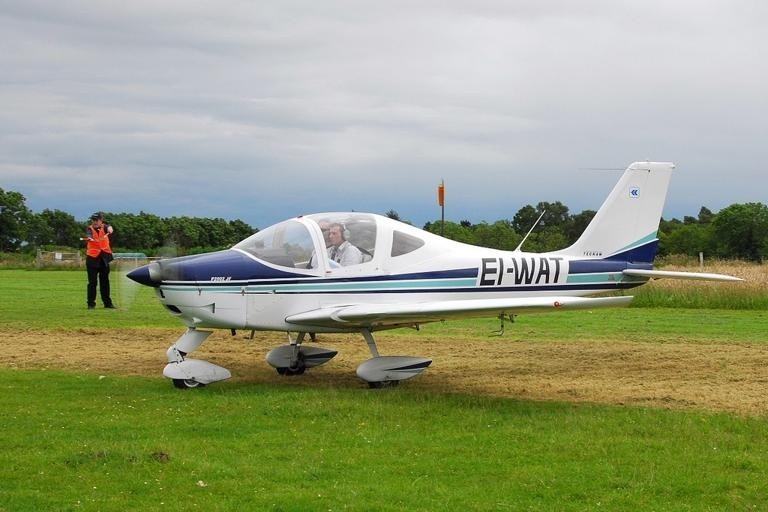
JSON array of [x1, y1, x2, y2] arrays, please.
[[88, 213, 101, 218]]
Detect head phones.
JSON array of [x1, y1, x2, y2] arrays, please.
[[328, 222, 351, 241]]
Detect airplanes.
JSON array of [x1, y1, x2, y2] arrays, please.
[[113, 158, 747, 391]]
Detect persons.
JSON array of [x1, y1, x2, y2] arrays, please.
[[308, 223, 362, 268], [311, 218, 332, 255], [85, 212, 117, 310]]
